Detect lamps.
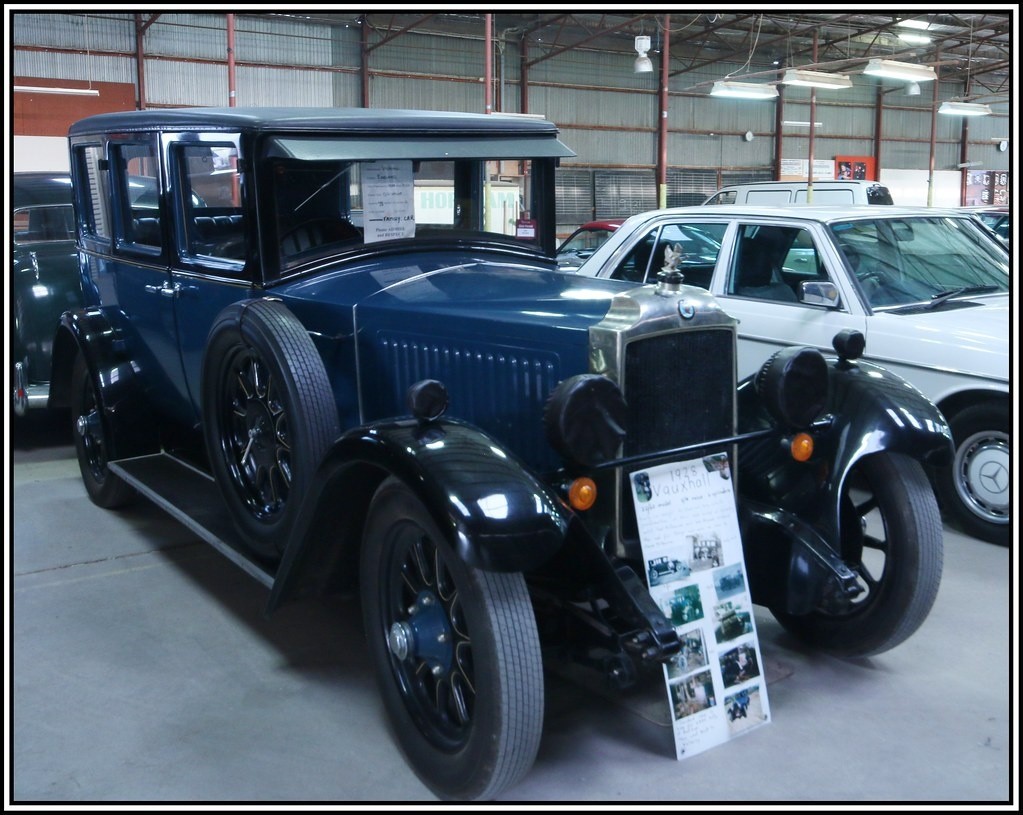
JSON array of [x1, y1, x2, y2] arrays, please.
[[782, 14, 854, 89], [709, 82, 779, 98], [863, 58, 937, 81], [937, 102, 992, 116], [14, 14, 99, 96], [634, 35, 654, 74], [779, 15, 824, 127], [903, 80, 921, 97]]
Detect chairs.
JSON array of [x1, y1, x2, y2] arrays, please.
[[816, 244, 886, 310], [716, 237, 796, 304]]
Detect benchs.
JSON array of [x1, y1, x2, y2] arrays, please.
[[131, 215, 242, 256], [15, 231, 75, 241], [619, 240, 781, 290]]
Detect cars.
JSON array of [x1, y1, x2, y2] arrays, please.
[[47, 105, 954, 801], [574, 203, 1013, 547], [9, 171, 209, 415], [553, 177, 1011, 308]]
